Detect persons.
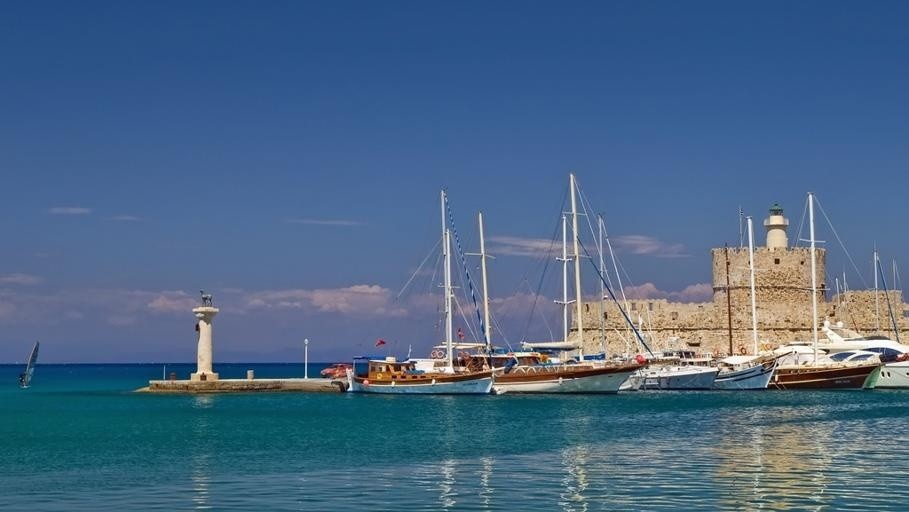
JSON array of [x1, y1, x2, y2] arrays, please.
[[793, 350, 800, 365], [16, 373, 25, 386]]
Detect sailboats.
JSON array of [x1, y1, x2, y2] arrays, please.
[[488, 174, 681, 395], [18, 341, 41, 388], [346, 185, 607, 395]]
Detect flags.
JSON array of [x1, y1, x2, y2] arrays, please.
[[458, 327, 465, 341]]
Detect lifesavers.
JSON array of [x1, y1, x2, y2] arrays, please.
[[456, 351, 464, 359], [713, 348, 719, 356], [431, 350, 438, 359], [436, 351, 444, 359], [765, 343, 773, 351], [462, 352, 470, 360], [740, 347, 747, 355]]
